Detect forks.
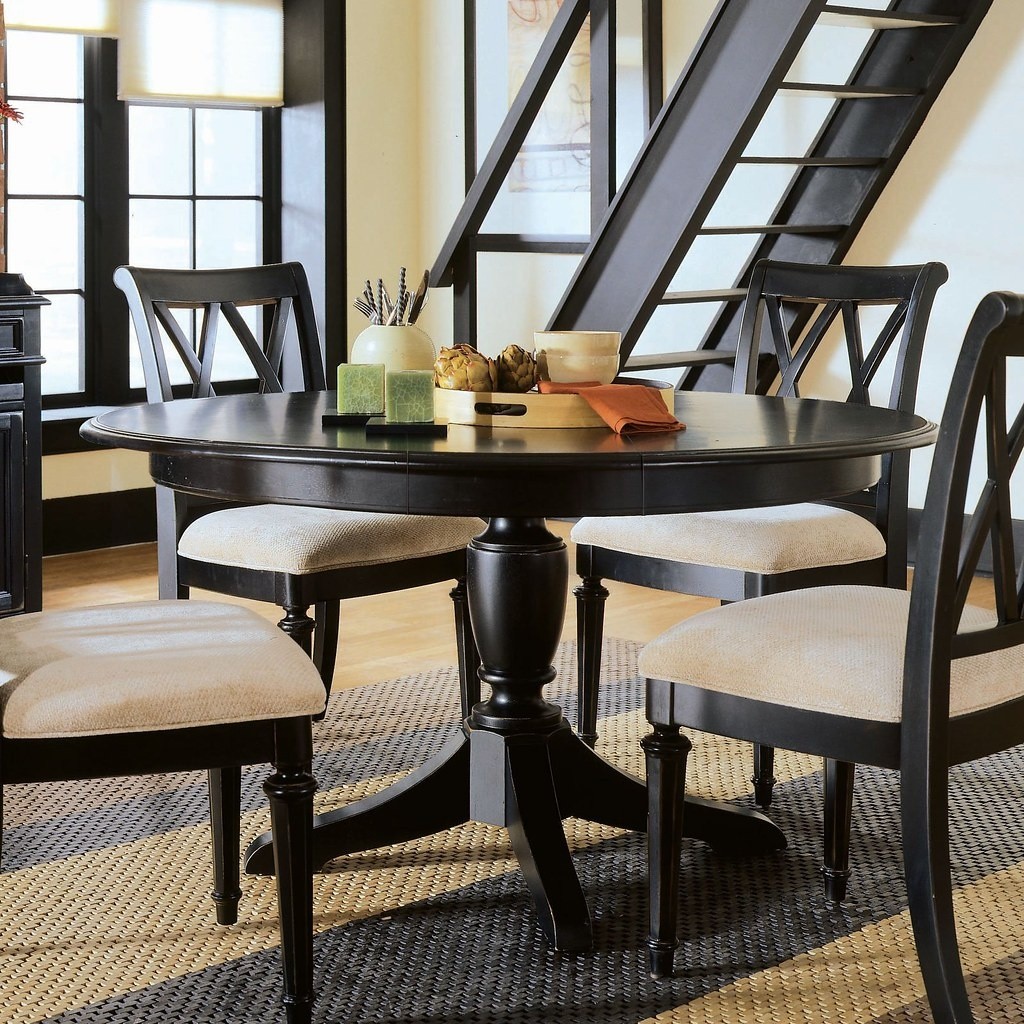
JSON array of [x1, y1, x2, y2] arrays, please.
[[353, 297, 377, 325]]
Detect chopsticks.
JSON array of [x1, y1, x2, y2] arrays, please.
[[363, 267, 410, 327]]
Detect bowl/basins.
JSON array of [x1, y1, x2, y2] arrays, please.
[[534, 331, 621, 384]]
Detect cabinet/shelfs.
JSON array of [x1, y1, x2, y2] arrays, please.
[[0, 273, 51, 619]]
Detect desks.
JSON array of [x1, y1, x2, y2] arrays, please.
[[77, 390, 939, 952]]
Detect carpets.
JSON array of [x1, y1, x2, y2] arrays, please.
[[1, 634, 1023, 1024]]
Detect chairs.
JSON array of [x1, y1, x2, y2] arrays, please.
[[570, 258, 948, 809], [0, 599, 327, 1024], [638, 290, 1024, 1024], [113, 260, 488, 729]]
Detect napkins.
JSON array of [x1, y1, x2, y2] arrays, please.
[[538, 381, 687, 434]]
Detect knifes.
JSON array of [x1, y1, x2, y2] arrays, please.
[[405, 270, 429, 326]]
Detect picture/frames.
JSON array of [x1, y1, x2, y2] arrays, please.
[[464, 0, 616, 253]]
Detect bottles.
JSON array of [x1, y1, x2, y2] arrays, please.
[[351, 325, 436, 370]]
